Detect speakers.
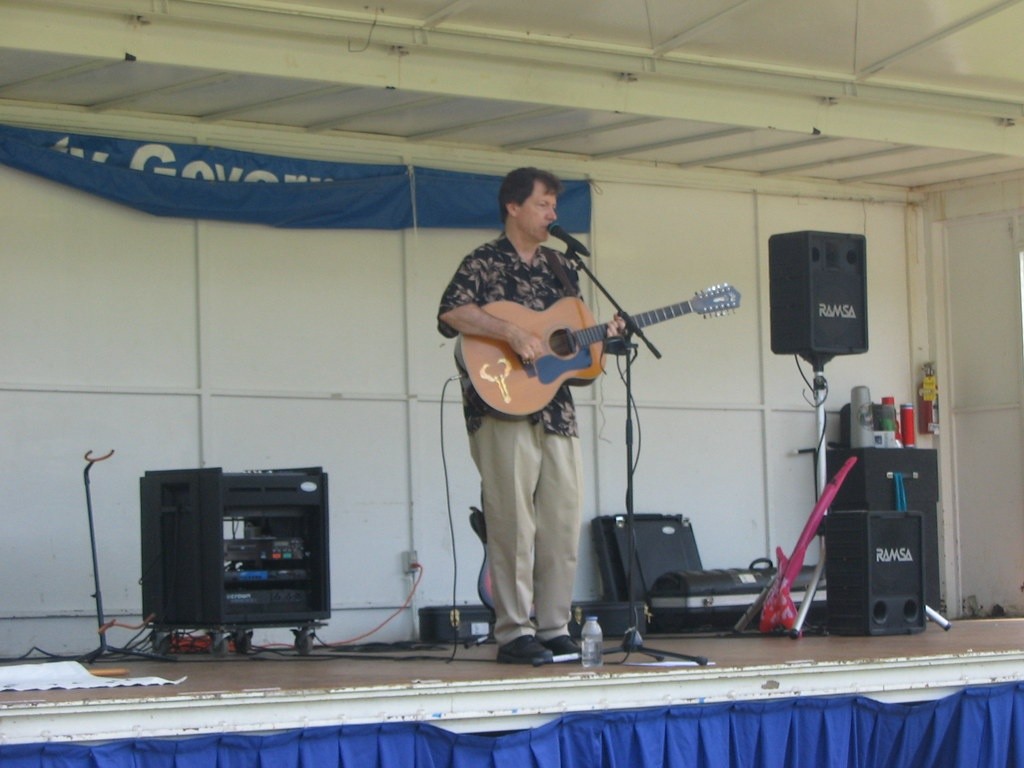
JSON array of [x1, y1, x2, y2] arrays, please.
[[825, 511, 927, 636], [767, 231, 868, 354]]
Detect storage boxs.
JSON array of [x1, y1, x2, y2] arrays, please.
[[826, 446, 939, 507]]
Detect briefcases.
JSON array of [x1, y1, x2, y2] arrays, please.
[[649, 558, 828, 632]]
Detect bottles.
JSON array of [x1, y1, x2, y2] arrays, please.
[[580, 616, 604, 668], [881, 397, 895, 431], [849, 386, 875, 449]]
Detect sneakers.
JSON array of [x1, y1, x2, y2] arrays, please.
[[498, 635, 553, 665], [542, 635, 582, 663]]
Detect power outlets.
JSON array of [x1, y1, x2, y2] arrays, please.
[[402, 550, 419, 573]]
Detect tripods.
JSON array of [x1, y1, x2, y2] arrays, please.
[[44, 449, 178, 666], [533, 249, 707, 667], [789, 352, 951, 639]]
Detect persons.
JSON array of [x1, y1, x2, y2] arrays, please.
[[436, 167, 626, 662]]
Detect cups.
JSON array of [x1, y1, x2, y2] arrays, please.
[[900, 403, 915, 448]]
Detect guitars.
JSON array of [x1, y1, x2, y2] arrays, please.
[[453, 281, 742, 422]]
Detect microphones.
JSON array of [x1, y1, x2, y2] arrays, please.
[[547, 223, 592, 258]]
